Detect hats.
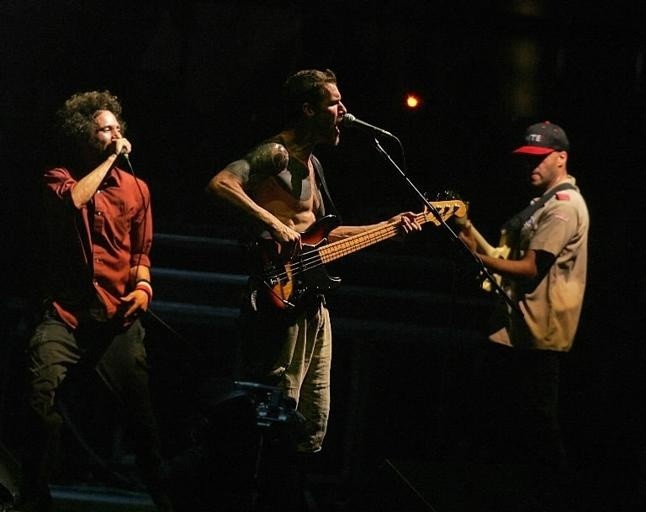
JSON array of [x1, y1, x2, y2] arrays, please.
[[510, 119, 573, 160]]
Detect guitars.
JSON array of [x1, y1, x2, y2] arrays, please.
[[443, 192, 512, 301], [252, 202, 465, 324]]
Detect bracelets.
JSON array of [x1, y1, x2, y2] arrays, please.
[[136, 280, 154, 302]]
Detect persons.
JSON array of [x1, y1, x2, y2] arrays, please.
[[457, 121, 590, 510], [206, 71, 424, 510], [25, 91, 175, 511]]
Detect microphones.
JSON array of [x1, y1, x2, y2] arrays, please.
[[342, 112, 393, 140], [113, 138, 131, 157]]
